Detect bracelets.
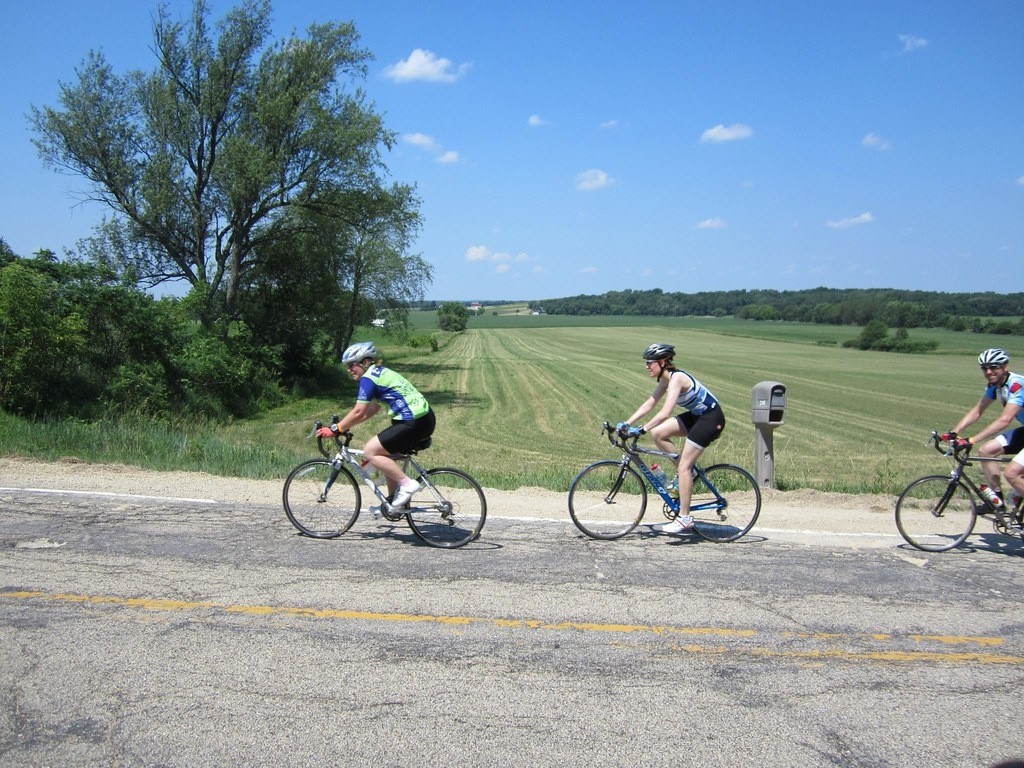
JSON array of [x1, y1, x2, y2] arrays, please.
[[970, 437, 975, 444], [338, 423, 345, 433]]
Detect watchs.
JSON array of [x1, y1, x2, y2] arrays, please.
[[638, 425, 646, 435], [329, 424, 342, 436]]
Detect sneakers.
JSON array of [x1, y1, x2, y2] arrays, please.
[[368, 506, 383, 517], [392, 479, 420, 507], [664, 481, 680, 499], [662, 516, 694, 534]]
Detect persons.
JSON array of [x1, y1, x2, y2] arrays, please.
[[315, 342, 436, 516], [616, 344, 726, 532], [941, 349, 1024, 515]]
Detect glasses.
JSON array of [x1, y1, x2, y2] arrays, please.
[[645, 360, 659, 367], [981, 364, 1004, 371], [349, 357, 365, 368]]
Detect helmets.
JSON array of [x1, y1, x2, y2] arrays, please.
[[978, 348, 1009, 365], [342, 342, 377, 366], [642, 343, 676, 361]]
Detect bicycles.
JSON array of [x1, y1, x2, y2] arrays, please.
[[895, 430, 1024, 552], [282, 414, 486, 549], [568, 422, 761, 543]]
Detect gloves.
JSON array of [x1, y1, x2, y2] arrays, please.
[[947, 437, 973, 455], [315, 423, 348, 437], [941, 431, 958, 442], [627, 427, 641, 437]]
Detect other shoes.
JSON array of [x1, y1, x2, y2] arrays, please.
[[974, 501, 1005, 515]]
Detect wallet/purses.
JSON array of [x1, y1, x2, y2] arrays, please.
[[616, 421, 629, 431]]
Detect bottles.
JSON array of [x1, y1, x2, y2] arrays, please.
[[360, 458, 383, 486], [979, 483, 1003, 508], [651, 463, 673, 490]]
